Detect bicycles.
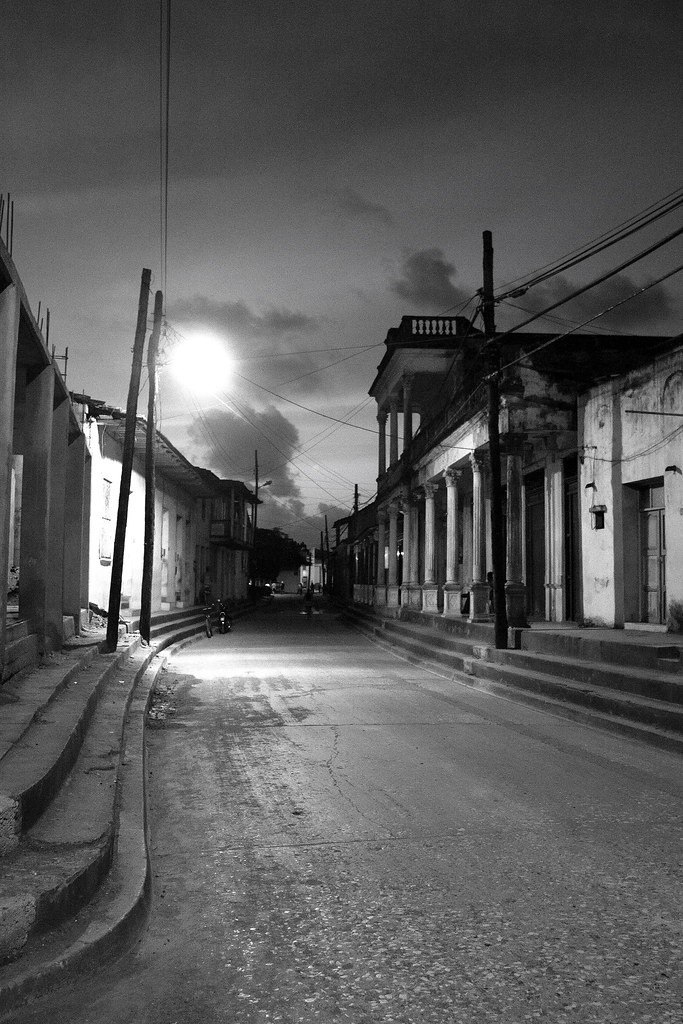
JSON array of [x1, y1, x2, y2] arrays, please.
[[203, 607, 215, 638]]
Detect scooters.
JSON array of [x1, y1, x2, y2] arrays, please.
[[218, 599, 233, 634]]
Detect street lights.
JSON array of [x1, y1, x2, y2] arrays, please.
[[138, 345, 216, 643], [250, 479, 272, 540]]
